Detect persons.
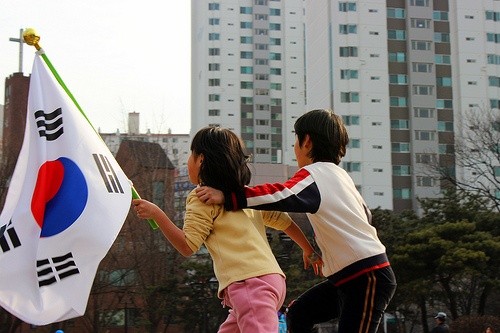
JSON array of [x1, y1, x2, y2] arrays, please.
[[195, 109, 398, 333], [132, 125, 324, 333], [430, 312, 450, 333]]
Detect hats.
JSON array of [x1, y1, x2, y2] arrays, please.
[[434, 312, 447, 318]]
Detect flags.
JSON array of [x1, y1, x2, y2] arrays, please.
[[0, 50, 132, 325]]
